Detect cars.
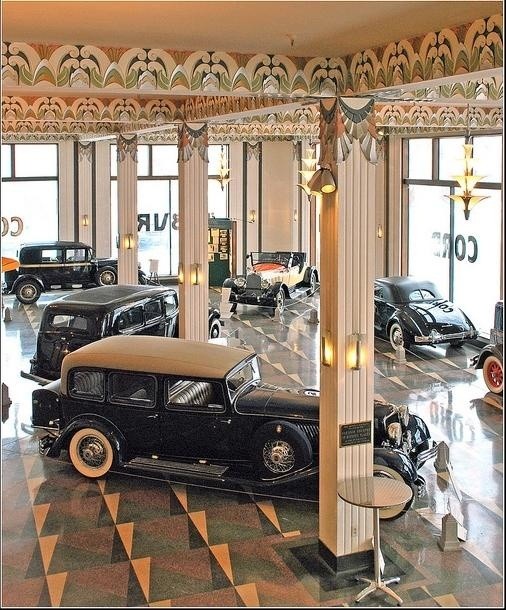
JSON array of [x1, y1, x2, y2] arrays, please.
[[20, 284, 225, 387], [223, 251, 320, 314], [470, 301, 503, 394], [374, 276, 480, 352], [31, 335, 438, 522], [5, 240, 146, 304]]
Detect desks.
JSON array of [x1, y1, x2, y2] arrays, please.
[[337, 477, 413, 606]]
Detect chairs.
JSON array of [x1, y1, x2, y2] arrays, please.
[[169, 380, 216, 406], [74, 371, 103, 395]]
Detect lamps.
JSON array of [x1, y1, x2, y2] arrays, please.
[[296, 136, 320, 202], [444, 104, 491, 221], [307, 167, 336, 198], [215, 142, 234, 191]]
